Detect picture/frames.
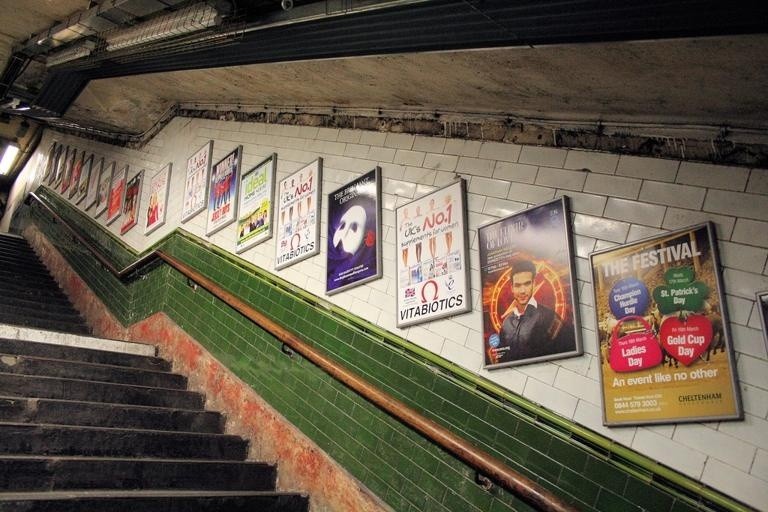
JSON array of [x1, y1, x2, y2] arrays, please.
[[182, 141, 214, 223], [475, 193, 586, 373], [233, 151, 279, 254], [586, 218, 746, 427], [203, 141, 245, 238], [324, 165, 385, 297], [43, 142, 172, 235], [272, 155, 325, 271], [392, 177, 475, 331]]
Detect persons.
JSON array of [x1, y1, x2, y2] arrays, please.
[[213, 173, 232, 211], [147, 193, 159, 226], [496, 261, 576, 362], [239, 210, 268, 238]]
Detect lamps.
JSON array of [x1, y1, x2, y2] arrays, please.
[[44, 40, 95, 67], [105, 3, 222, 53], [0, 142, 21, 176]]
